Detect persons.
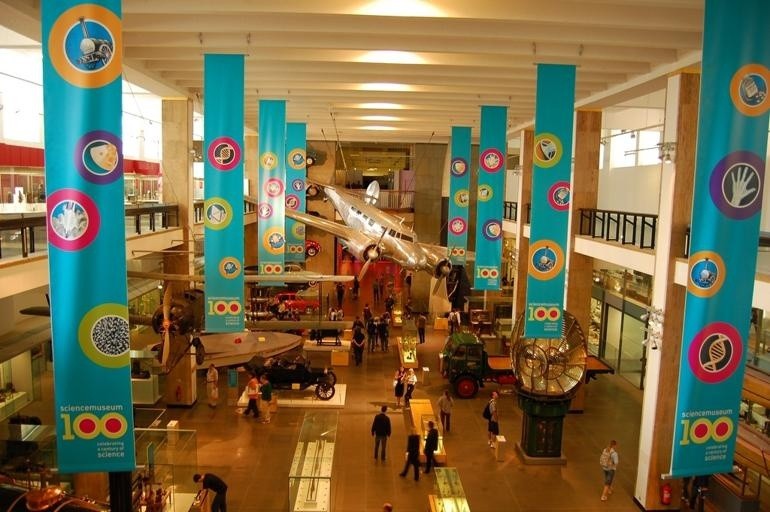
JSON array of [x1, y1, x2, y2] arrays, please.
[[369, 366, 453, 482], [240, 374, 258, 419], [259, 373, 273, 425], [273, 254, 461, 363], [493, 313, 503, 335], [383, 503, 393, 512], [602, 440, 619, 499], [511, 278, 514, 287], [502, 273, 507, 285], [207, 362, 219, 408], [488, 390, 499, 449], [193, 473, 227, 511]]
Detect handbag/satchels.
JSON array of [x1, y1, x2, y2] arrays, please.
[[392, 380, 398, 387], [248, 390, 259, 400], [211, 387, 219, 402]]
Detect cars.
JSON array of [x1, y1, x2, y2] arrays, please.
[[244, 262, 321, 316], [304, 182, 320, 198], [305, 238, 321, 258], [306, 150, 318, 170]]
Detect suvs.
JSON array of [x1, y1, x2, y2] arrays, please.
[[241, 356, 338, 401]]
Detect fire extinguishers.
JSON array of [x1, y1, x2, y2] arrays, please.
[[662, 483, 672, 505], [176, 386, 181, 401]]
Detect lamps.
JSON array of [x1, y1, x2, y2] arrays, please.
[[657, 141, 675, 163]]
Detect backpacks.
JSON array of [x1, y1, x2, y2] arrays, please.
[[482, 399, 494, 420], [600, 448, 615, 468]]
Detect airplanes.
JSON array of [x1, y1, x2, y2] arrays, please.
[[16, 269, 360, 364], [242, 175, 512, 287]]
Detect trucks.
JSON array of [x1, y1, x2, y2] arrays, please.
[[436, 327, 620, 401]]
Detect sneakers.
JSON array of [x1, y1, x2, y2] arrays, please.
[[243, 411, 270, 424], [399, 472, 405, 476], [601, 487, 613, 501], [488, 440, 496, 449], [423, 471, 430, 473], [208, 404, 216, 409]]
